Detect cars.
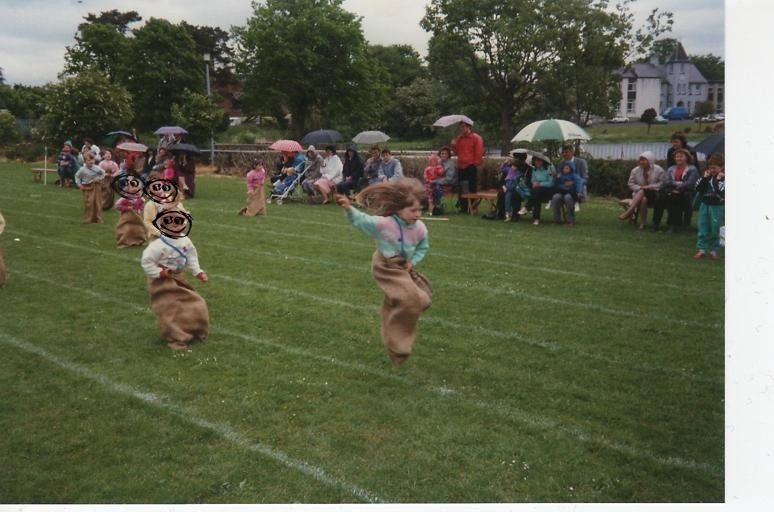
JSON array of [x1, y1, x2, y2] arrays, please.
[[651, 114, 668, 124], [578, 117, 592, 127], [608, 115, 630, 123], [694, 112, 725, 123]]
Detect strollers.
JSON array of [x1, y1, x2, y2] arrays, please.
[[266, 158, 314, 205]]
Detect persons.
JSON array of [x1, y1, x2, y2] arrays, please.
[[423, 152, 446, 216], [428, 147, 457, 215], [270, 148, 433, 366], [54, 134, 208, 352], [619, 122, 725, 258], [450, 121, 483, 215], [239, 159, 267, 216], [496, 145, 589, 226]]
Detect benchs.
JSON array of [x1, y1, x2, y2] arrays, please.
[[32, 168, 57, 182], [461, 192, 580, 221], [320, 182, 368, 204], [619, 199, 706, 224]]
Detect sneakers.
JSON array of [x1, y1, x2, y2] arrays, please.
[[575, 201, 580, 211], [690, 250, 719, 260], [545, 200, 552, 210], [504, 209, 574, 226]]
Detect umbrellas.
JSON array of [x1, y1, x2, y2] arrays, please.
[[432, 112, 474, 128], [352, 131, 391, 150], [269, 139, 303, 152], [153, 126, 188, 134], [301, 127, 344, 147], [107, 130, 133, 136], [511, 114, 592, 176]]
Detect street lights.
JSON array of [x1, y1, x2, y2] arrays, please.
[[202, 51, 215, 168]]
